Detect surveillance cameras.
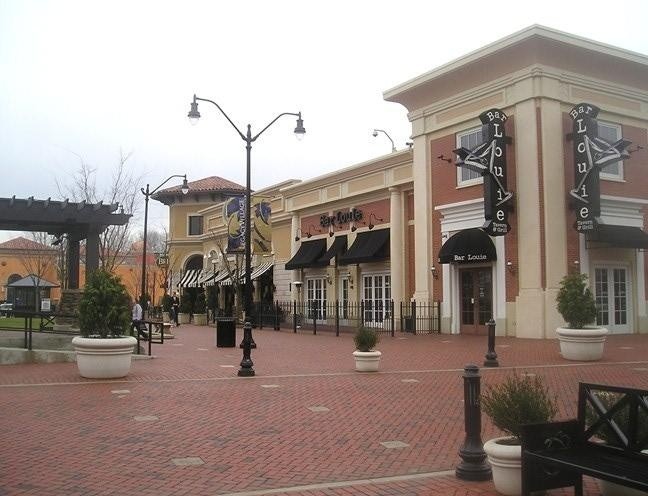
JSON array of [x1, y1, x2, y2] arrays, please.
[[373, 131, 377, 137]]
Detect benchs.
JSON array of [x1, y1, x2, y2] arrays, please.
[[520, 382, 648, 495]]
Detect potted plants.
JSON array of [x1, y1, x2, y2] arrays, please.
[[574, 382, 648, 495], [475, 367, 563, 495], [177, 294, 191, 324], [352, 323, 382, 373], [72, 264, 137, 379], [159, 293, 174, 323], [554, 272, 608, 361], [138, 293, 151, 320], [192, 292, 208, 326]]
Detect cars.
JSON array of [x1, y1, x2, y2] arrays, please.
[[0, 303, 12, 316]]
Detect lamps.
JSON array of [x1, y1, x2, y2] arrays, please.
[[295, 213, 384, 241], [507, 260, 516, 274], [573, 260, 581, 270], [347, 271, 353, 284], [326, 273, 332, 285], [430, 264, 439, 280], [372, 129, 396, 152]]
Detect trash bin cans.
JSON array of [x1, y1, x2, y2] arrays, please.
[[215, 317, 238, 348], [53, 299, 60, 308], [40, 299, 51, 311]]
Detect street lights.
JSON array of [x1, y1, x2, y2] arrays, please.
[[184, 90, 310, 380], [140, 172, 190, 319], [372, 127, 398, 154]]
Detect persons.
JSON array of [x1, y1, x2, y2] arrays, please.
[[6, 297, 12, 318], [130, 298, 149, 339], [147, 299, 150, 310], [169, 291, 181, 326]]
